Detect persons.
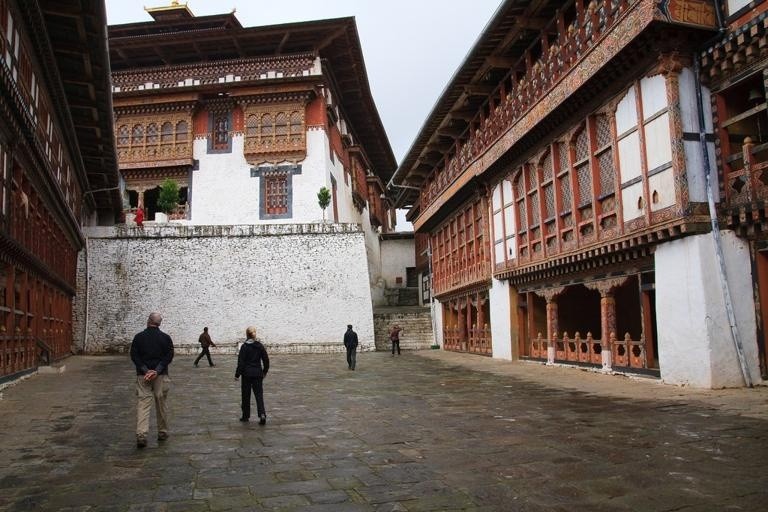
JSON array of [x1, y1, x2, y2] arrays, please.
[[130, 313, 174, 447], [344, 325, 358, 370], [233, 327, 269, 424], [390, 325, 401, 356], [194, 326, 217, 367], [119, 211, 126, 223], [134, 206, 147, 229]]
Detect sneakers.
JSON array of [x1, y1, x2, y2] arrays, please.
[[137, 437, 146, 446], [241, 415, 248, 421], [259, 414, 266, 425], [158, 433, 169, 441]]
